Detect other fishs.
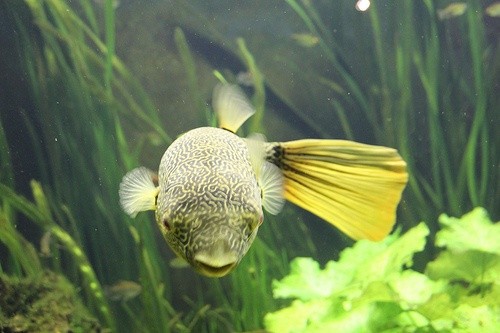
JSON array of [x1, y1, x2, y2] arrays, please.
[[118, 83, 408, 278]]
